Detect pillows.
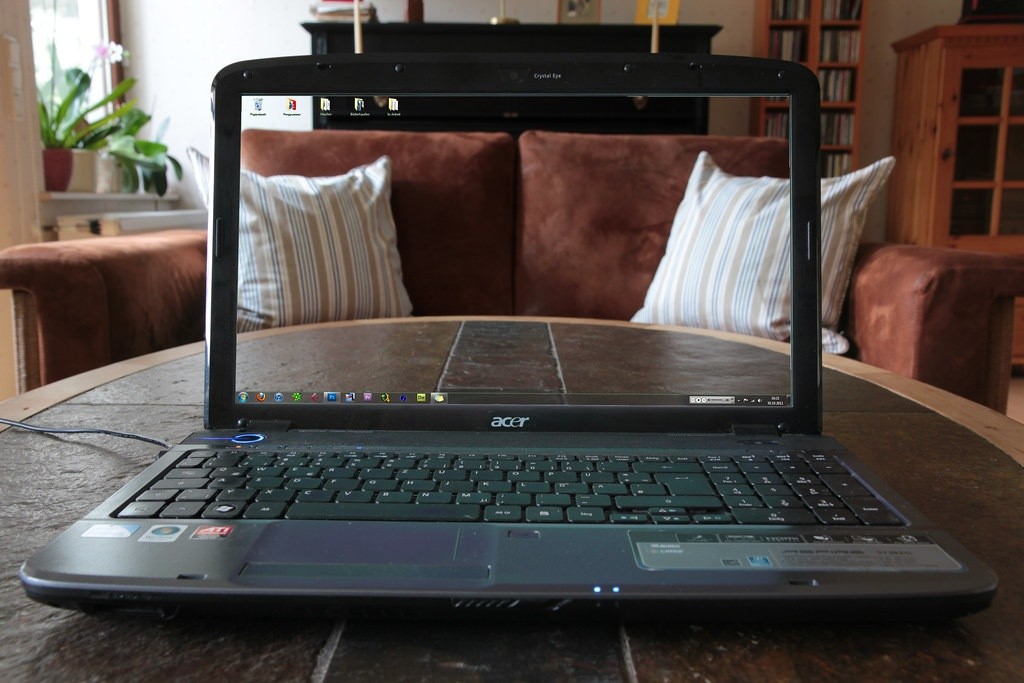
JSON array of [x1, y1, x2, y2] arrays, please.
[[186, 146, 413, 334], [626, 149, 896, 354]]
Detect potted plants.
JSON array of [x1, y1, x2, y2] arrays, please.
[[72, 125, 122, 193]]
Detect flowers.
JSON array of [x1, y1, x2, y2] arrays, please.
[[39, 41, 138, 147]]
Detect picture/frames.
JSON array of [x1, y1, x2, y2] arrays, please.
[[634, 0, 681, 25], [557, 0, 601, 24]]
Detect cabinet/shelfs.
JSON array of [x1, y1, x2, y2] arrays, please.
[[882, 23, 1024, 364], [748, 0, 869, 171]]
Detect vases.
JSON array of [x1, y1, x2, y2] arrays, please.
[[43, 149, 74, 192]]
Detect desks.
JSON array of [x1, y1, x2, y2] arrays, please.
[[0, 315, 1021, 680]]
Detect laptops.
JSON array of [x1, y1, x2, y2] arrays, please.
[[17, 52, 1002, 632]]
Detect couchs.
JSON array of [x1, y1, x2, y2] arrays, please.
[[0, 126, 1020, 416]]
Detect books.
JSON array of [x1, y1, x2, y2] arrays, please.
[[309, 0, 376, 22], [767, 1, 861, 178]]
